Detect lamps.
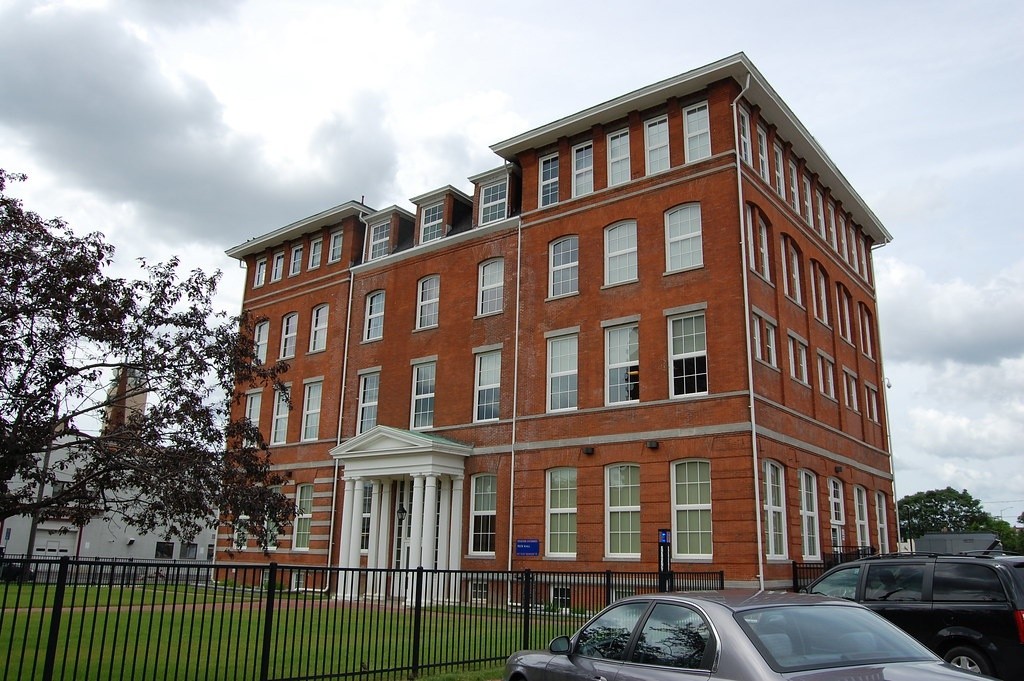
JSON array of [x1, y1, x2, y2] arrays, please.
[[397, 503, 407, 521]]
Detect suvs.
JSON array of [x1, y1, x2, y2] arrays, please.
[[795, 547, 1023, 681]]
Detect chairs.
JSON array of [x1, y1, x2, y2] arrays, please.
[[872, 570, 900, 600]]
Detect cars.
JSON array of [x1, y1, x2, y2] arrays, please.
[[960, 547, 1024, 588], [503, 587, 1005, 681]]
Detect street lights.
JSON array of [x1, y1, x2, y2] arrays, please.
[[998, 506, 1015, 521], [902, 504, 914, 553]]
